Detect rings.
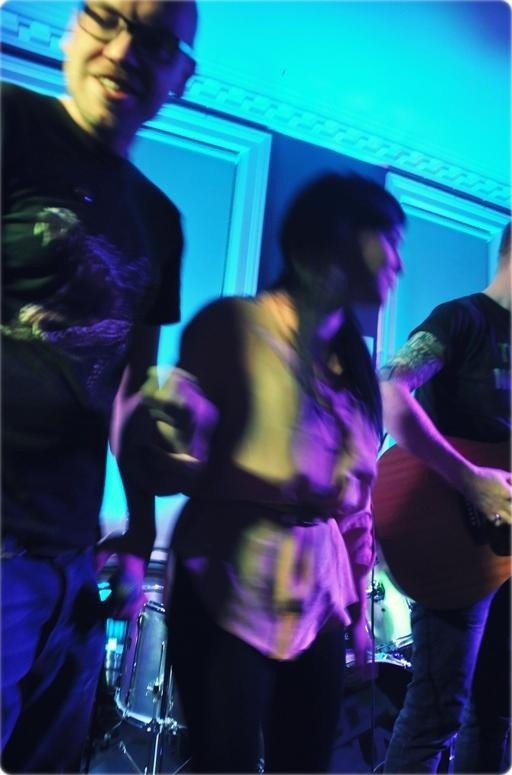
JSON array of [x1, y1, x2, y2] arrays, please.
[[494, 513, 501, 521]]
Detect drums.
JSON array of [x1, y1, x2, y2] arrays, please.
[[331, 652, 456, 772], [114, 585, 188, 736]]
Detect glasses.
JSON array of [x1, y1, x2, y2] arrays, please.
[[78, 0, 198, 65]]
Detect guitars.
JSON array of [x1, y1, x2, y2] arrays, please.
[[372, 436, 511, 610]]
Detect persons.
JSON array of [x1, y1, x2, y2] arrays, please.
[[375, 222, 511, 775], [0, 0, 185, 775], [163, 172, 408, 775]]
